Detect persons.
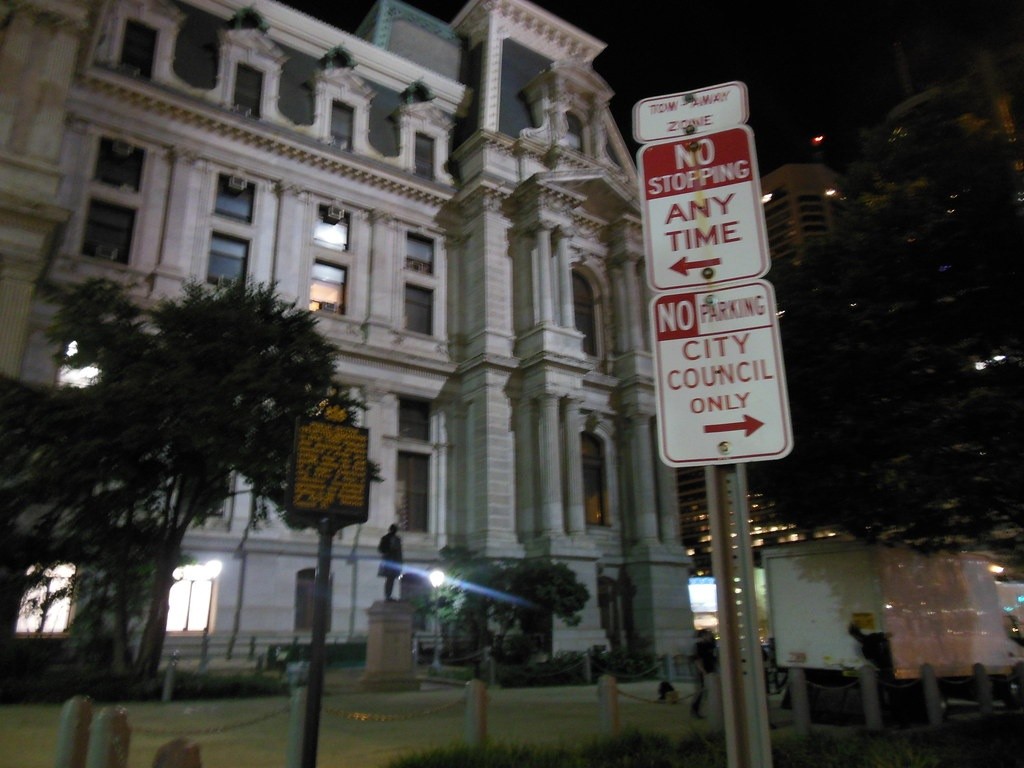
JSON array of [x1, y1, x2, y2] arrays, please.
[[688, 629, 777, 730], [847, 624, 896, 727], [378, 524, 403, 602], [126, 609, 147, 665]]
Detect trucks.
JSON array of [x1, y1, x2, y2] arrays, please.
[[760, 540, 1024, 721]]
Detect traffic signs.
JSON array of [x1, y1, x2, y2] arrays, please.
[[636, 127, 770, 292], [649, 279, 793, 467]]
